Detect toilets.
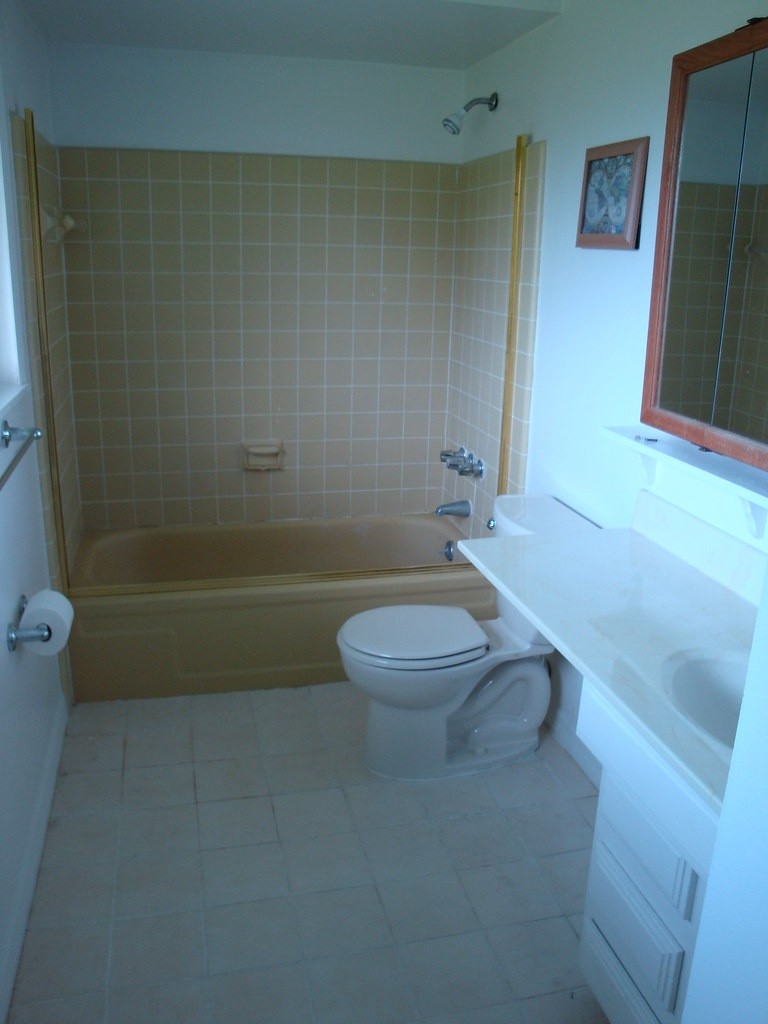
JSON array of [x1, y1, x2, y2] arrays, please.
[[334, 494, 603, 780]]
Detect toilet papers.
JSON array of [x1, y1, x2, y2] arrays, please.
[[18, 589, 75, 657]]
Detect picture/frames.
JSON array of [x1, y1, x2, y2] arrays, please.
[[575, 137, 650, 250]]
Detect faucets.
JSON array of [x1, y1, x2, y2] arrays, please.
[[434, 499, 472, 518]]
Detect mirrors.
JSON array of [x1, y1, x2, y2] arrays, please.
[[640, 17, 768, 475]]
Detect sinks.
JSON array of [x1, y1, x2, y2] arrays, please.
[[657, 647, 756, 751]]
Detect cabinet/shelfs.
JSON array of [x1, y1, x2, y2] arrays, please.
[[574, 679, 717, 1024]]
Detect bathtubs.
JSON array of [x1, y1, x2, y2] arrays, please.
[[59, 513, 500, 703]]
